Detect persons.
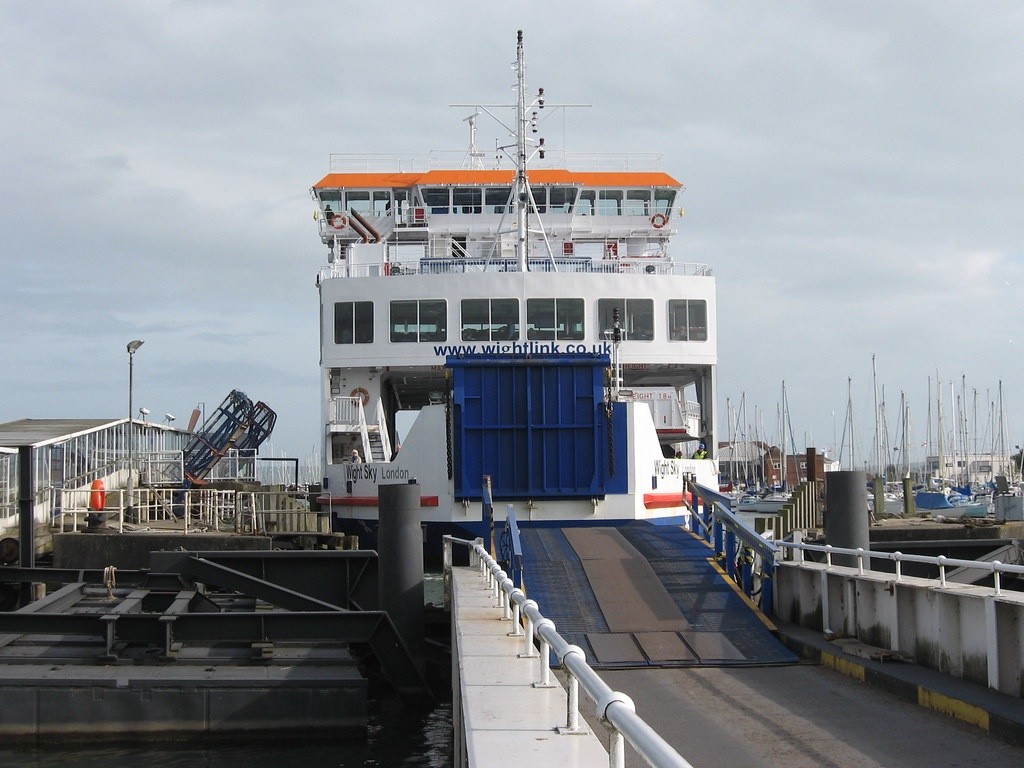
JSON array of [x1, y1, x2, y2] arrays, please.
[[692, 443, 707, 459], [389, 445, 401, 461], [350, 449, 362, 464]]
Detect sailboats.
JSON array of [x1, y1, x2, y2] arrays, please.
[[718, 353, 1024, 520]]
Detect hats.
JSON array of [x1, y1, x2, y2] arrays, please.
[[699, 444, 705, 449]]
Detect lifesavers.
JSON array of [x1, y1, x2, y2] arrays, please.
[[330, 215, 347, 229], [650, 213, 667, 228], [349, 388, 369, 407], [90, 479, 107, 511]]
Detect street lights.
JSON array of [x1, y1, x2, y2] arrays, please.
[[126, 339, 145, 523]]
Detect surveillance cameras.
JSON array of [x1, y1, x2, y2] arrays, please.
[[165, 414, 176, 421], [140, 408, 150, 415]]
